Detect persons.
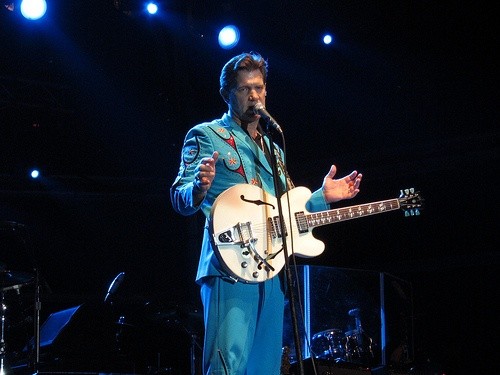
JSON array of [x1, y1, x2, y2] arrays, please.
[[170, 53, 362, 375]]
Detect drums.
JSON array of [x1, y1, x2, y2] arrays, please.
[[344, 331, 373, 361], [312, 329, 345, 360]]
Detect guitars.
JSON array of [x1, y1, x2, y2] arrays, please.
[[208, 184, 422, 285]]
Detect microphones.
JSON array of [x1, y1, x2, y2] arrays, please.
[[253, 102, 283, 134]]
